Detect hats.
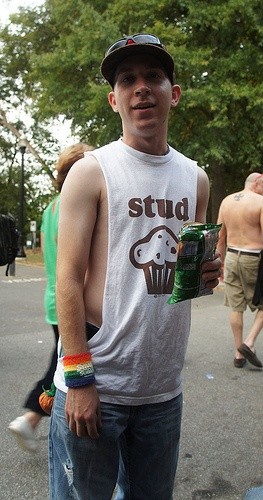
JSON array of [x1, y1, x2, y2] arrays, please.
[[100, 34, 174, 87]]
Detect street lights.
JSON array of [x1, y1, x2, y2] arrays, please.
[[16, 137, 28, 260]]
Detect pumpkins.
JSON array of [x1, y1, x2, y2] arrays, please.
[[39, 389, 54, 414]]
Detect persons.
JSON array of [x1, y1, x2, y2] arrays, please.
[[9, 143, 100, 452], [48, 34, 221, 500], [213, 173, 263, 368]]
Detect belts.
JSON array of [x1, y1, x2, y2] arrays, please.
[[227, 248, 259, 257]]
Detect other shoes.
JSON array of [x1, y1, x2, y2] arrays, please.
[[238, 343, 262, 367], [7, 416, 38, 453], [234, 357, 246, 368]]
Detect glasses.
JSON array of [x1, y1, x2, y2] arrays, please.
[[104, 33, 163, 58]]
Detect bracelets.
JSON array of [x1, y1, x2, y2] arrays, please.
[[61, 355, 96, 388]]
